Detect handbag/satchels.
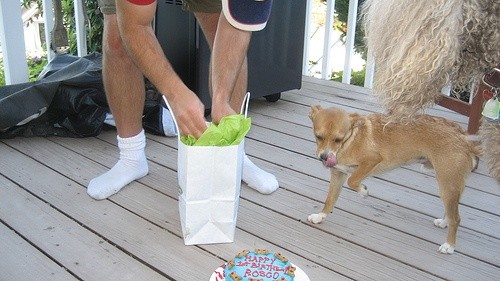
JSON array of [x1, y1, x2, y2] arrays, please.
[[161, 92, 251, 246]]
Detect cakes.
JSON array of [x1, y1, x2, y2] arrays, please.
[[212, 248, 308, 281]]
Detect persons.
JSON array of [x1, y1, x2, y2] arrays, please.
[[87, 0, 279, 201]]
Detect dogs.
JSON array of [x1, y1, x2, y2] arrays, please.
[[306, 104, 482, 256]]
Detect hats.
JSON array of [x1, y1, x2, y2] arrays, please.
[[221, 0, 273, 32]]
